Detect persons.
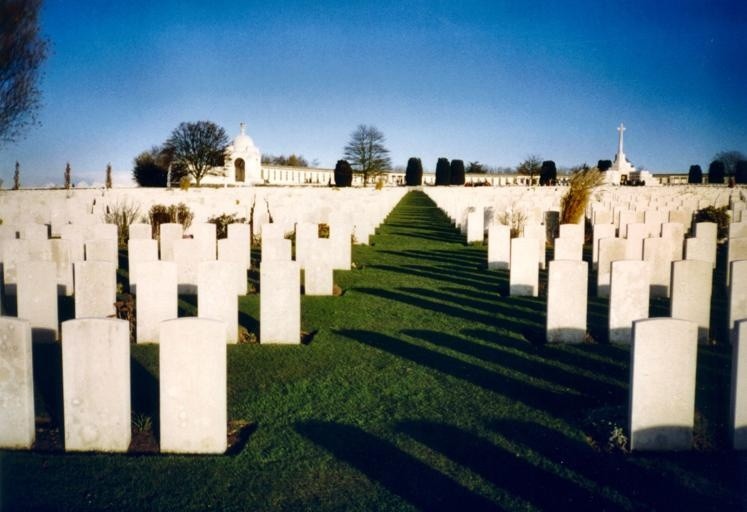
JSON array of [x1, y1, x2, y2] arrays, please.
[[622, 178, 645, 186], [726, 175, 734, 188]]
